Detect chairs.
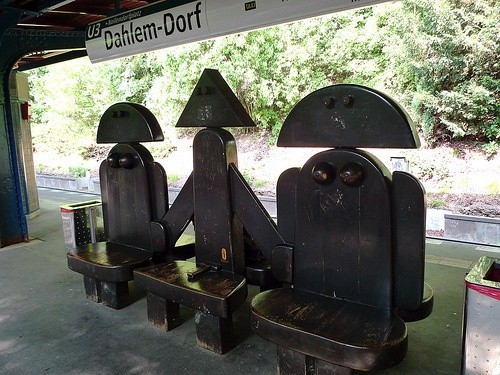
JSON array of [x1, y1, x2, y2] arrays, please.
[[66, 68, 434, 375]]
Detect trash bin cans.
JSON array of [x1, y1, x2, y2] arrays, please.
[[462, 255, 500, 374], [59, 200, 102, 252]]
